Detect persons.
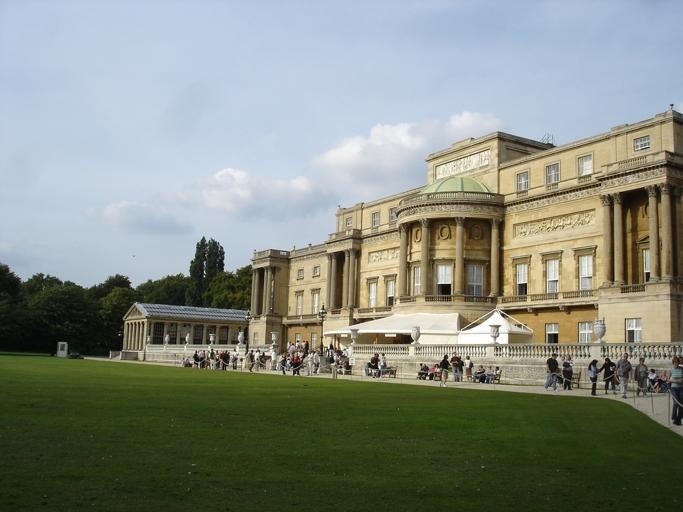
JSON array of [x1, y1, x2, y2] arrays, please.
[[181, 348, 239, 371], [367, 353, 380, 369], [245, 349, 267, 372], [379, 353, 386, 377], [416, 352, 501, 384], [271, 349, 277, 370], [544, 353, 683, 425], [278, 339, 351, 379]]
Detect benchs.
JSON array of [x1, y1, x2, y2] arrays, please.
[[485, 370, 502, 384], [363, 365, 398, 378], [558, 371, 581, 388]]
